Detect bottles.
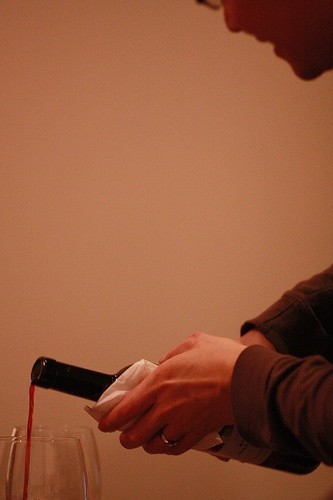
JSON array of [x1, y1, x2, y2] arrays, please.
[[29, 356, 322, 476]]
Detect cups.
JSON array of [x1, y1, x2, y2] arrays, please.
[[0, 436, 87, 500], [5, 426, 101, 500]]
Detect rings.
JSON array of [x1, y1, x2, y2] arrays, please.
[[161, 431, 176, 447]]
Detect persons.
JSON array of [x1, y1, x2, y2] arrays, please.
[[98, 0, 333, 466]]
